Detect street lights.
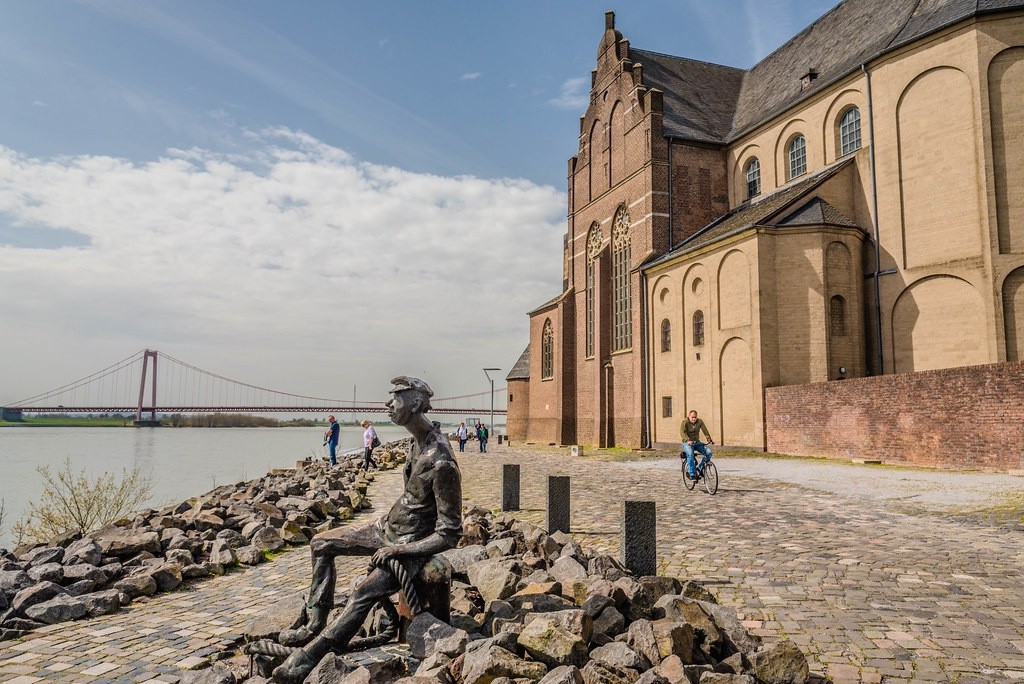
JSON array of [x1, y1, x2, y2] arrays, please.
[[483, 368, 501, 437]]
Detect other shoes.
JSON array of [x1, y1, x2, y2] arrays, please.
[[696, 467, 704, 477], [689, 475, 698, 483]]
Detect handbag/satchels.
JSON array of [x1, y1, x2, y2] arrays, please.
[[371, 437, 381, 449]]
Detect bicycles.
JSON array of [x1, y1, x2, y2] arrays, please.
[[681, 442, 719, 495]]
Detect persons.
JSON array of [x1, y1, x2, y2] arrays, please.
[[325, 415, 340, 466], [475, 421, 482, 441], [478, 423, 488, 453], [271, 376, 463, 683], [457, 422, 469, 452], [360, 420, 378, 471], [679, 410, 715, 483]]
